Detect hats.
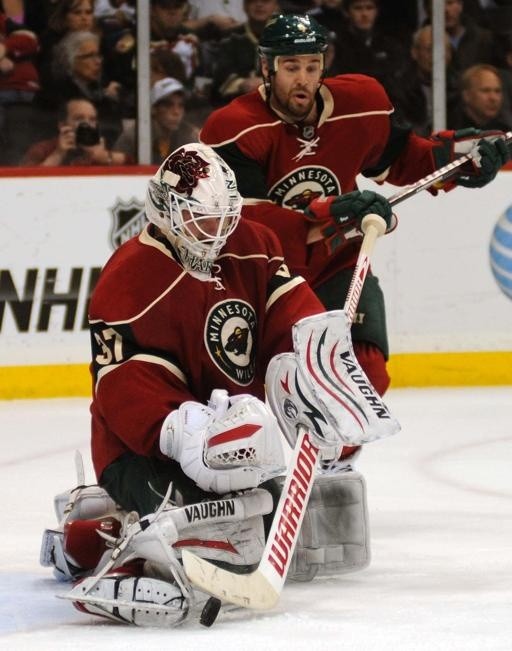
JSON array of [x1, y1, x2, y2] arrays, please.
[[151, 77, 185, 106], [4, 30, 39, 56]]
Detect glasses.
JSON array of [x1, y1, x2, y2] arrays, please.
[[75, 50, 105, 62]]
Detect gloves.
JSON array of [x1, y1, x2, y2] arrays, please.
[[429, 127, 511, 188], [302, 189, 394, 232]]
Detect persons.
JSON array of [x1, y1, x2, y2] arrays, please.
[[2, 2, 512, 170], [34, 143, 403, 631], [196, 11, 512, 475]]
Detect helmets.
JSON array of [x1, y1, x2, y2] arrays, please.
[[256, 12, 330, 76], [145, 142, 244, 272]]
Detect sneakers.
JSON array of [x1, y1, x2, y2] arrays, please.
[[82, 575, 190, 627]]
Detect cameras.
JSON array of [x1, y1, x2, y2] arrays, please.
[[74, 122, 100, 146]]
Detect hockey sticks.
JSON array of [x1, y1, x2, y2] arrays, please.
[[183, 213, 388, 608]]
